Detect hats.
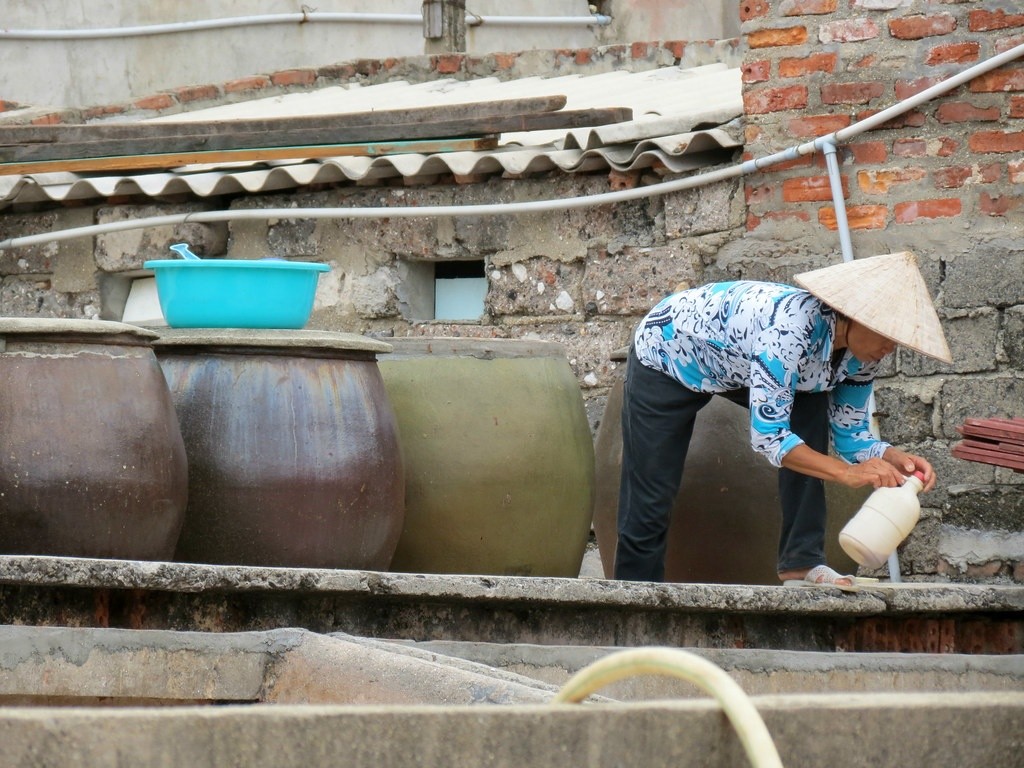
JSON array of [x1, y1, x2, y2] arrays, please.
[[792, 251, 951, 363]]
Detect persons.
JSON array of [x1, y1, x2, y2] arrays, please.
[[613, 251, 953, 594]]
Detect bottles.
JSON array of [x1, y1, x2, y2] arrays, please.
[[839, 468, 928, 569]]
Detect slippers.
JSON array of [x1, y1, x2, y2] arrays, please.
[[783, 564, 858, 593]]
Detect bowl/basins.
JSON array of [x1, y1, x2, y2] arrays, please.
[[143, 258, 332, 329]]
